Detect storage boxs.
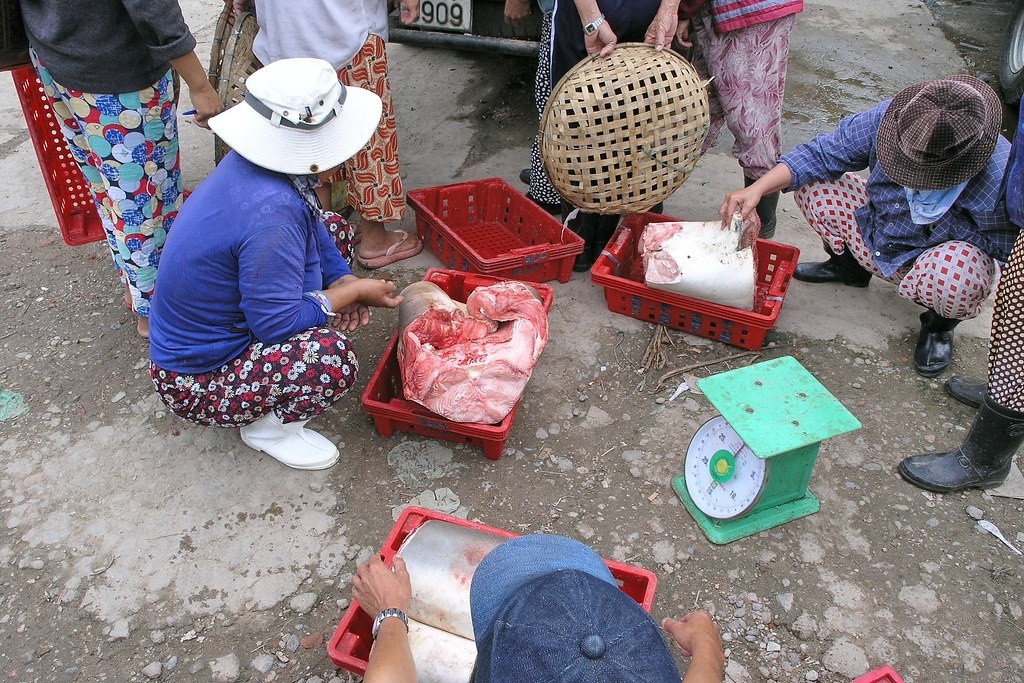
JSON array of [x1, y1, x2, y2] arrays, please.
[[10, 63, 131, 245], [325, 178, 799, 683]]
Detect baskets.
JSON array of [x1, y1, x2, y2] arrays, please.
[[208, 1, 264, 167], [539, 40, 710, 215]]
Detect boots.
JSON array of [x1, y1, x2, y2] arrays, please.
[[897, 393, 1024, 494], [562, 201, 621, 272], [945, 375, 989, 409], [792, 241, 873, 288], [239, 411, 340, 471], [744, 174, 781, 240], [648, 199, 664, 214], [913, 298, 964, 378]]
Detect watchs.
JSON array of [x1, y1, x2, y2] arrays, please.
[[582, 13, 605, 35], [372, 607, 409, 640]]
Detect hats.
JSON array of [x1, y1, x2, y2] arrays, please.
[[877, 74, 1002, 191], [207, 57, 382, 174], [468, 533, 683, 683]]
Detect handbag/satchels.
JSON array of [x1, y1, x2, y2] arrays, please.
[[0, 0, 33, 71]]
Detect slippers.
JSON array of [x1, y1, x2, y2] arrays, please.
[[351, 223, 423, 270]]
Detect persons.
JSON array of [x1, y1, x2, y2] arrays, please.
[[234, 0, 422, 268], [351, 533, 723, 683], [718, 74, 1020, 377], [670, 0, 802, 238], [550, 0, 664, 272], [898, 94, 1024, 492], [21, 0, 222, 337], [149, 58, 403, 470], [505, 0, 560, 213]]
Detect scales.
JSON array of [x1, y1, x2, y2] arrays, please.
[[672, 356, 862, 546]]
